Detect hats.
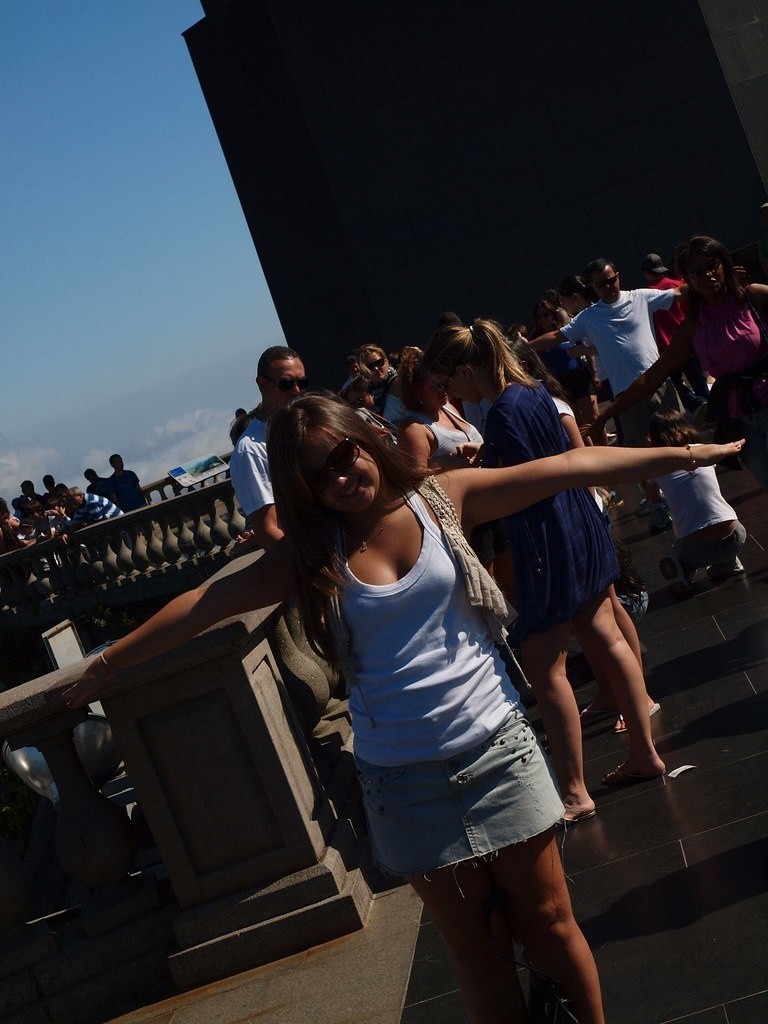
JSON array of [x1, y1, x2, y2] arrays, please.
[[641, 253, 669, 273]]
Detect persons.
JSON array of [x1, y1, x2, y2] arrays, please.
[[0, 454, 147, 574], [62, 395, 745, 1024], [230, 347, 327, 633], [339, 236, 768, 829], [230, 406, 261, 446]]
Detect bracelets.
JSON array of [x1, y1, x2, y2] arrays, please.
[[100, 653, 122, 672], [686, 445, 695, 475]]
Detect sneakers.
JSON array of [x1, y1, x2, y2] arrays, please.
[[658, 551, 693, 597], [648, 505, 672, 534], [707, 555, 745, 577]]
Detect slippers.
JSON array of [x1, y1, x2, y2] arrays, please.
[[602, 762, 666, 784], [615, 702, 661, 733], [579, 702, 619, 726], [553, 802, 596, 832]]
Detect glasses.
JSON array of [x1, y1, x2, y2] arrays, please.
[[264, 374, 309, 393], [353, 397, 364, 407], [534, 310, 549, 320], [366, 357, 385, 370], [595, 273, 617, 289], [300, 438, 361, 495], [686, 258, 725, 279]]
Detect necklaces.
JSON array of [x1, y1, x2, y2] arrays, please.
[[342, 512, 390, 550]]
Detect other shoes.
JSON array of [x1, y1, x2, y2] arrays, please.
[[632, 499, 669, 516]]
